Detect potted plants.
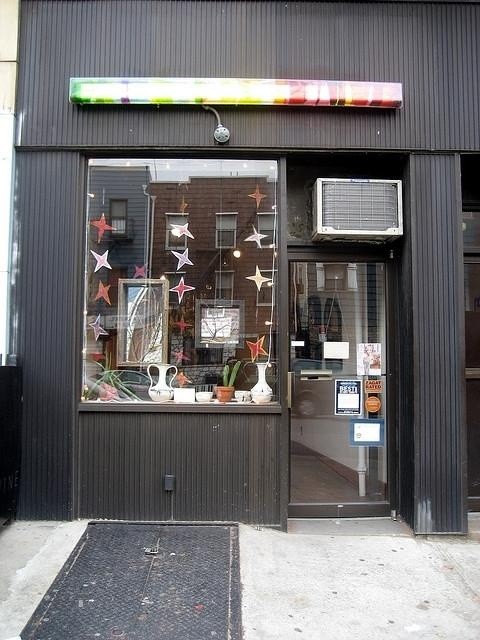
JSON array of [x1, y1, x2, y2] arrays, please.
[[214, 361, 241, 402], [81, 350, 157, 402]]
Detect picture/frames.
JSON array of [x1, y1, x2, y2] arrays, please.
[[194, 299, 246, 349], [117, 278, 169, 367]]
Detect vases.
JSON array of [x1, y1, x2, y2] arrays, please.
[[147, 363, 178, 402], [250, 363, 273, 402]]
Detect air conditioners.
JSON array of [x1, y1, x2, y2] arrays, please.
[[311, 178, 404, 243]]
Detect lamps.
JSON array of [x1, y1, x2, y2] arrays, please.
[[69, 76, 404, 144]]
